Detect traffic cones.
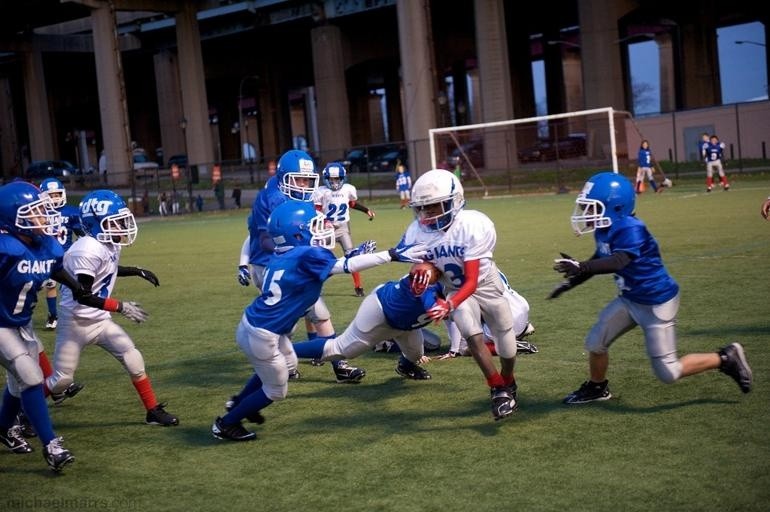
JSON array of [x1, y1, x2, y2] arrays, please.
[[172, 166, 180, 179], [211, 166, 222, 184], [268, 157, 277, 175]]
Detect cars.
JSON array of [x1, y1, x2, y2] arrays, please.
[[446, 141, 484, 168], [26, 159, 83, 178], [169, 154, 188, 168], [373, 149, 407, 171], [519, 132, 589, 164]]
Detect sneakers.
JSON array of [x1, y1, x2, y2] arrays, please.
[[400, 203, 412, 209], [723, 342, 752, 394], [355, 286, 364, 297], [515, 323, 539, 353], [706, 182, 730, 192], [212, 395, 265, 442], [51, 382, 84, 407], [490, 378, 519, 420], [562, 381, 612, 403], [145, 401, 179, 426], [288, 341, 431, 383], [41, 436, 75, 471], [0, 409, 37, 453], [45, 315, 59, 329], [655, 187, 664, 193]]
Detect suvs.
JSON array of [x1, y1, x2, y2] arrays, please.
[[332, 143, 390, 173]]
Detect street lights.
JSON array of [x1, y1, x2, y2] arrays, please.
[[735, 39, 765, 48]]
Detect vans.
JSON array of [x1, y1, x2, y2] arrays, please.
[[99, 141, 158, 181]]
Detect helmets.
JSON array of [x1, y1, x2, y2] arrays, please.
[[574, 172, 636, 228], [78, 189, 129, 240], [266, 200, 321, 255], [408, 169, 466, 233], [0, 177, 65, 237], [276, 150, 320, 202], [322, 162, 346, 191]]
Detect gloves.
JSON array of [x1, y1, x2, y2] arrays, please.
[[426, 296, 454, 325], [388, 234, 429, 264], [72, 282, 99, 302], [554, 252, 588, 278], [367, 210, 375, 222], [408, 269, 431, 297], [116, 300, 151, 324], [545, 279, 576, 300], [344, 240, 377, 260], [136, 269, 160, 287], [431, 350, 461, 361], [238, 265, 252, 286]]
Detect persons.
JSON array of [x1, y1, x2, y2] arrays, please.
[[761, 195, 770, 222], [698, 132, 732, 192], [211, 147, 538, 443], [543, 171, 754, 405], [634, 139, 664, 194], [0, 164, 205, 474]]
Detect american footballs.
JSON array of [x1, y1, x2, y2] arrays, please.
[[409, 263, 441, 284]]
